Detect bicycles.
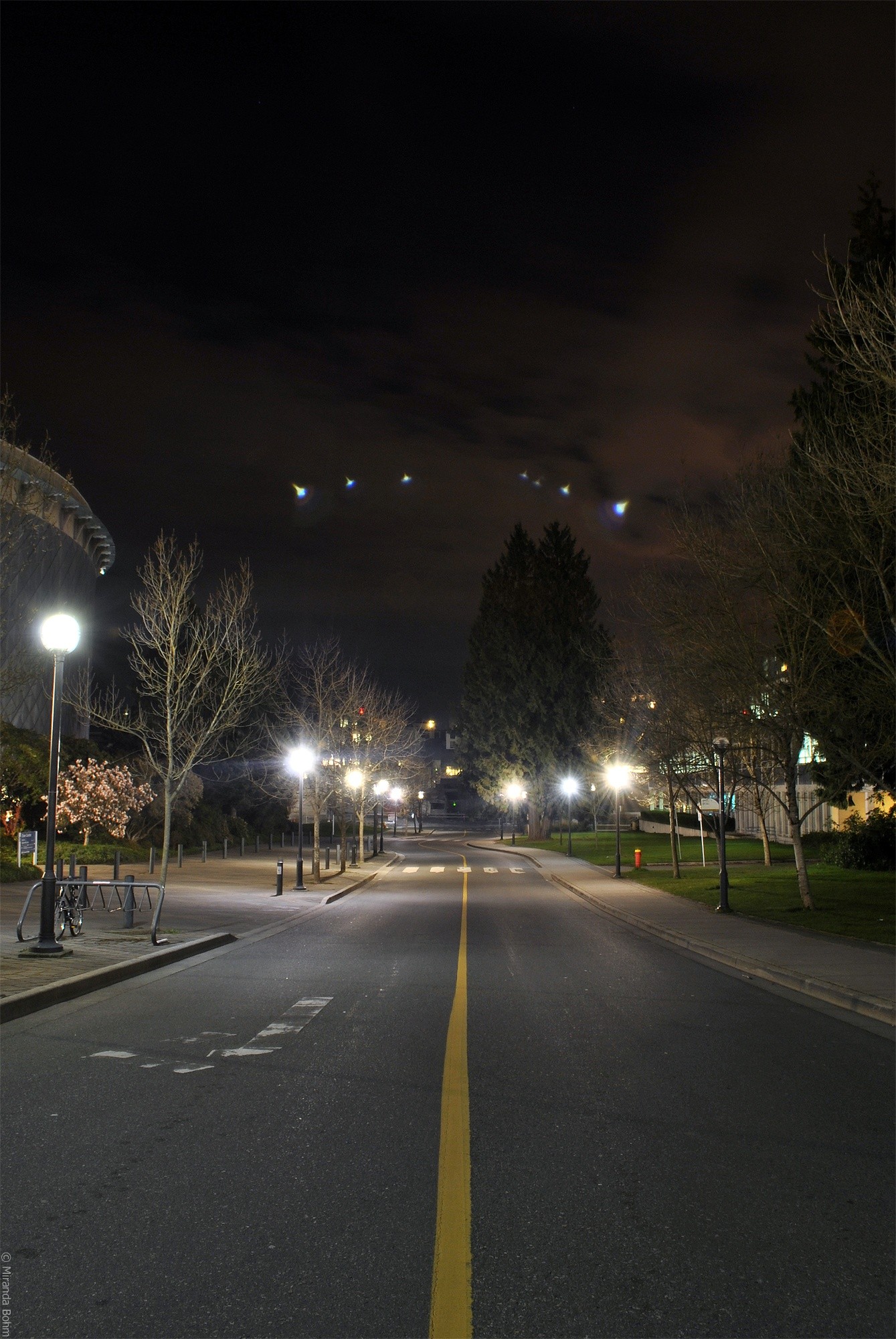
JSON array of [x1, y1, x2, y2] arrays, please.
[[53, 875, 86, 941]]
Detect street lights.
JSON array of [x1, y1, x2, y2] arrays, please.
[[375, 779, 389, 854], [388, 786, 402, 837], [604, 765, 630, 877], [711, 735, 733, 911], [27, 613, 82, 954], [507, 783, 520, 845], [285, 745, 315, 890], [345, 769, 363, 867], [417, 793, 424, 830], [560, 777, 579, 857]]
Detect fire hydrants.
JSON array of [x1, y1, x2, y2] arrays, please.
[[634, 848, 642, 869]]
